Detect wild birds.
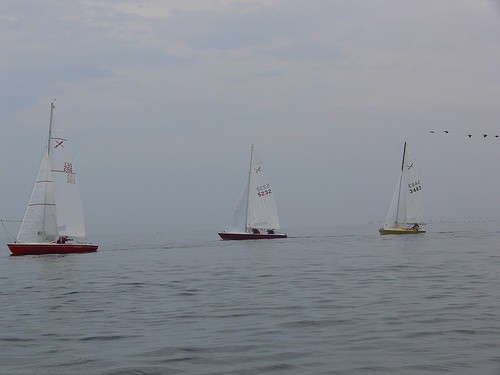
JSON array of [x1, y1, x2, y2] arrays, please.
[[467, 133, 473, 138], [442, 130, 449, 134], [482, 133, 488, 138], [493, 135, 500, 138], [429, 131, 435, 133]]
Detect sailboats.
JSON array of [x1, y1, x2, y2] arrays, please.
[[217, 144, 288, 241], [377, 141, 432, 234], [6, 95, 101, 256]]
